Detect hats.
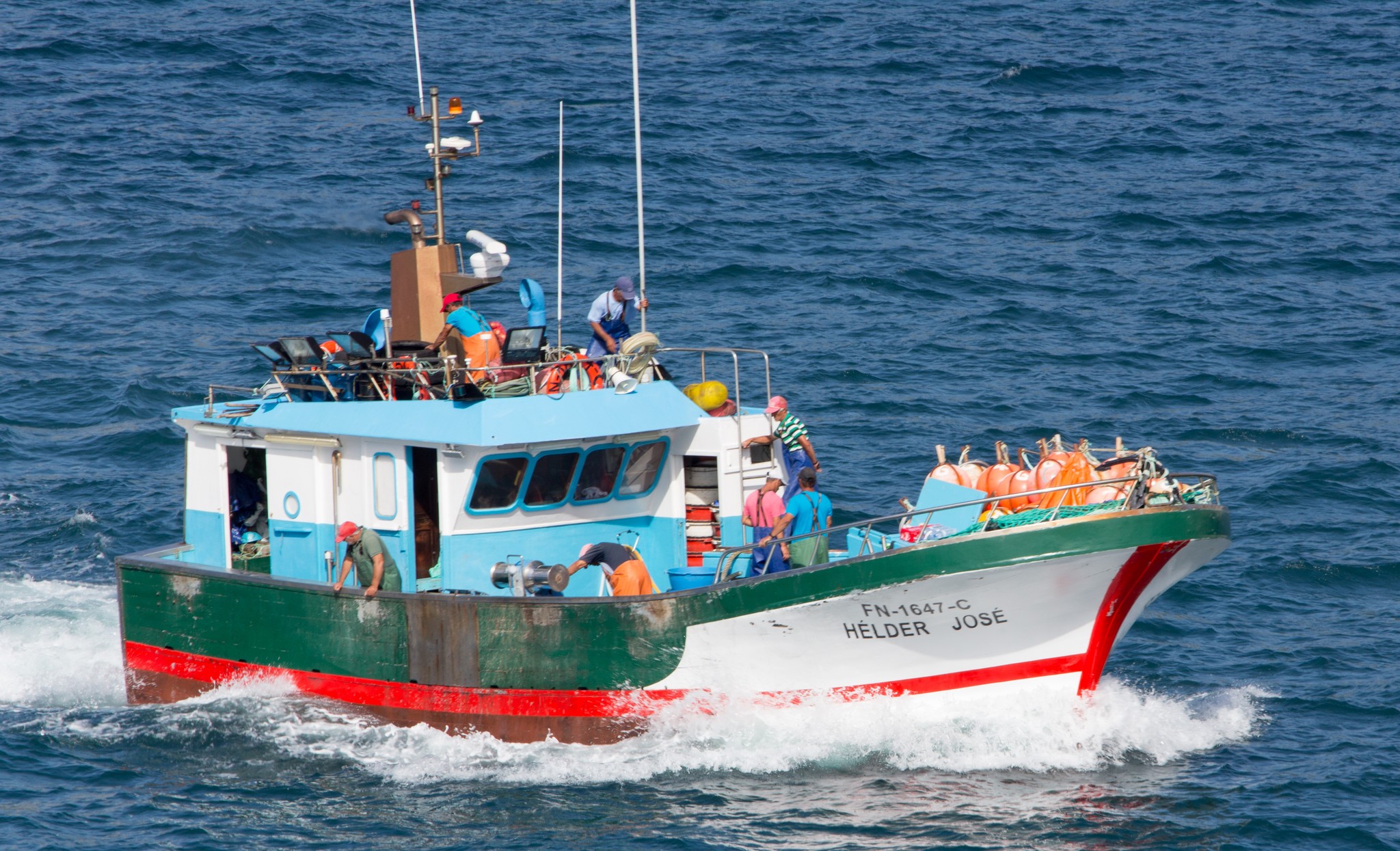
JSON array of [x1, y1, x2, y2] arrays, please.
[[766, 470, 789, 485], [796, 467, 817, 482], [335, 521, 358, 543], [579, 544, 593, 557], [440, 293, 463, 313], [764, 396, 787, 414], [615, 277, 635, 299]]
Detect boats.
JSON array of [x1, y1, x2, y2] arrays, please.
[[115, 0, 1254, 744]]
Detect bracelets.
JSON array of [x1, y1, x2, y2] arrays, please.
[[812, 460, 818, 465], [769, 533, 774, 538]]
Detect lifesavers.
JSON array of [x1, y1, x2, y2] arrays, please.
[[311, 340, 345, 371], [381, 355, 431, 400], [545, 353, 602, 397]]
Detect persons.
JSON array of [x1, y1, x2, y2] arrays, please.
[[565, 542, 653, 597], [741, 395, 822, 545], [330, 521, 402, 599], [740, 467, 789, 576], [575, 275, 649, 390], [758, 467, 834, 571], [423, 293, 500, 387]]
